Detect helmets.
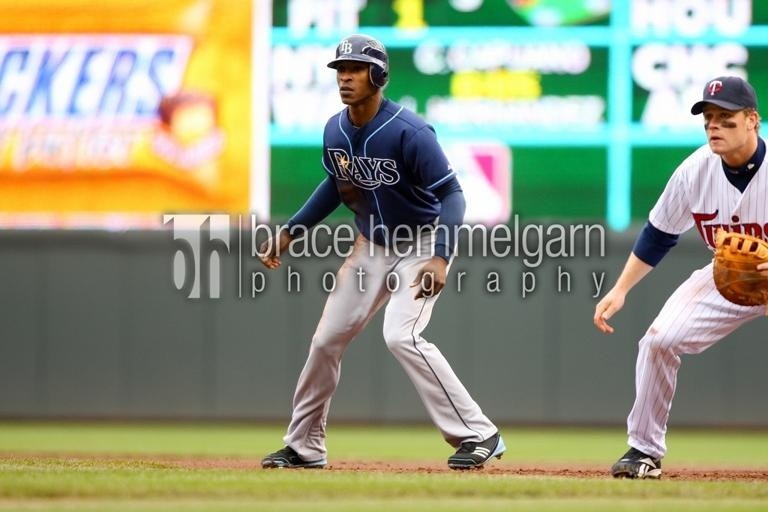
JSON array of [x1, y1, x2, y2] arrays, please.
[[326, 34, 389, 88]]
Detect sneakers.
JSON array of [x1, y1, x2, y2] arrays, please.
[[447, 430, 506, 469], [260, 446, 328, 468], [612, 447, 661, 479]]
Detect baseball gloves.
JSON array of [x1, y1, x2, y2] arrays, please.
[[714, 226, 767, 306]]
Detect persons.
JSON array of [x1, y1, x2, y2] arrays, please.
[[258, 34, 506, 470], [593, 75, 767, 477]]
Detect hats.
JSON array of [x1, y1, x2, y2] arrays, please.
[[690, 78, 755, 115]]
